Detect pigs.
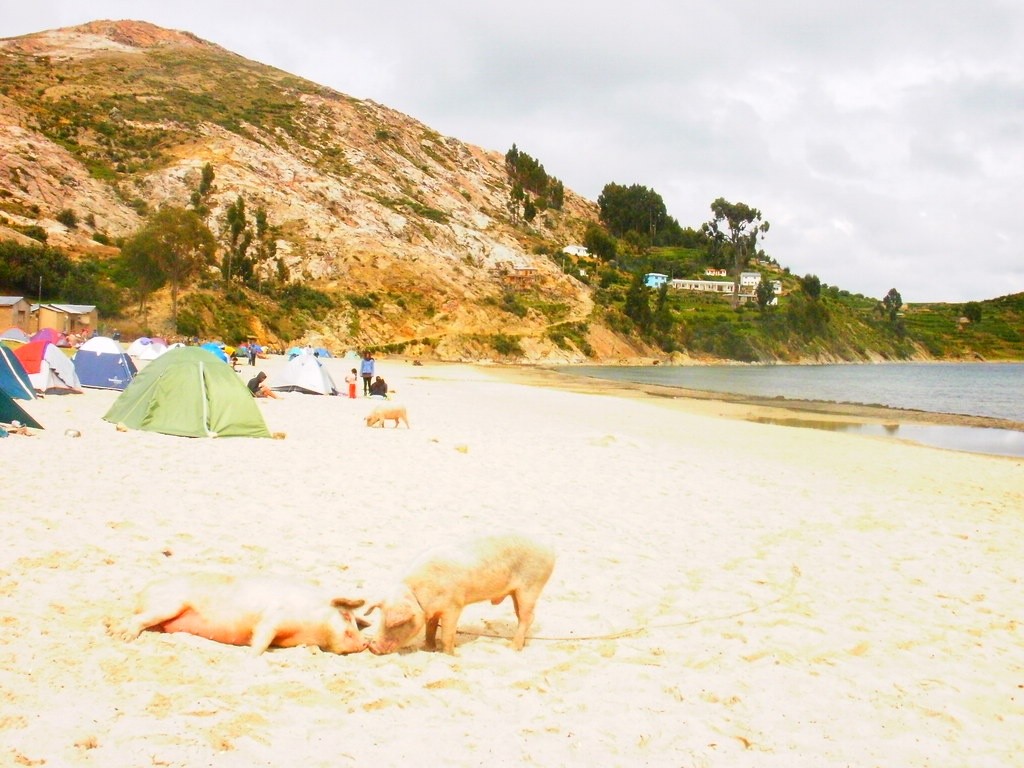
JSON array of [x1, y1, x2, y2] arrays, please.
[[363, 529, 555, 656], [104, 571, 370, 657], [364, 405, 410, 429]]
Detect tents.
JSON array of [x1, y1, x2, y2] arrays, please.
[[67, 336, 140, 392], [101, 345, 274, 440], [126, 336, 185, 360], [200, 342, 269, 364], [285, 347, 360, 362], [0, 325, 82, 431], [268, 353, 342, 396]]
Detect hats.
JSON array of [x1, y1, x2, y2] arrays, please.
[[376, 376, 381, 381]]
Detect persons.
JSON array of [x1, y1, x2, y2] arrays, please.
[[247, 347, 252, 366], [182, 334, 199, 346], [228, 357, 241, 374], [251, 344, 257, 366], [246, 371, 282, 399], [80, 325, 121, 345], [359, 352, 375, 397], [267, 347, 286, 356], [369, 376, 388, 399], [345, 367, 357, 399]]
